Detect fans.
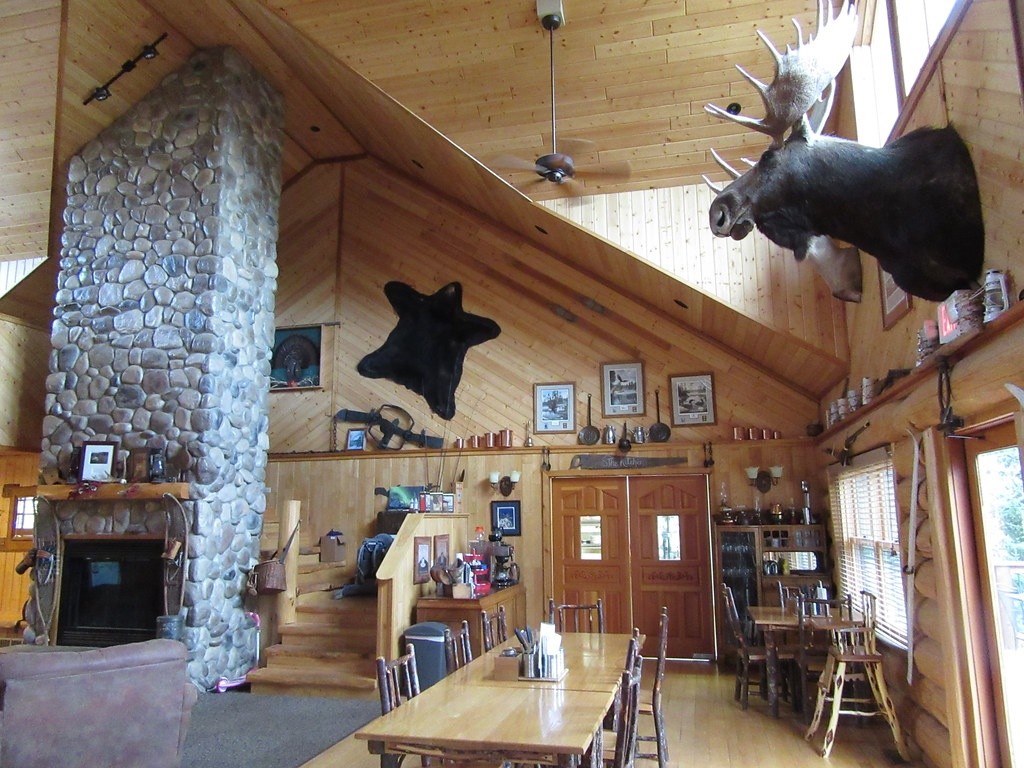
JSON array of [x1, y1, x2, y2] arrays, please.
[[475, 14, 631, 207]]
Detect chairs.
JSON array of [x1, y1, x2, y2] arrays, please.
[[720, 583, 863, 728], [374, 598, 669, 768]]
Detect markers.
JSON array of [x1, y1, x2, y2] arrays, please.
[[527, 628, 533, 643], [515, 628, 529, 649]]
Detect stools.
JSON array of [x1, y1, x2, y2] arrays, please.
[[807, 591, 913, 763]]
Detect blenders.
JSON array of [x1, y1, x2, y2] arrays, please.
[[491, 542, 519, 587]]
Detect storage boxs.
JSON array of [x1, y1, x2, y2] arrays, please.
[[316, 535, 347, 563], [451, 470, 465, 514]]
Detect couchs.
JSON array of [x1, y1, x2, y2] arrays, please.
[[0, 638, 198, 768]]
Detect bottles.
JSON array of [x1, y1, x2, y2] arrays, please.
[[474, 525, 486, 541], [634, 427, 645, 443]]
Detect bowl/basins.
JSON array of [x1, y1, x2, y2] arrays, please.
[[806, 424, 823, 435], [771, 512, 784, 524]]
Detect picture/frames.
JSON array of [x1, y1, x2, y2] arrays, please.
[[877, 261, 914, 332], [346, 428, 367, 451], [491, 500, 521, 537], [533, 382, 577, 435], [433, 534, 449, 568], [599, 359, 647, 419], [79, 441, 119, 484], [414, 536, 432, 586], [668, 371, 718, 429]]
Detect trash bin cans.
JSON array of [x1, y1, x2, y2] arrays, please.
[[403, 621, 449, 693]]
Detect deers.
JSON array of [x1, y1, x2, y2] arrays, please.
[[698, 0, 983, 303]]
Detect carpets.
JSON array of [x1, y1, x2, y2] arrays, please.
[[178, 690, 385, 768]]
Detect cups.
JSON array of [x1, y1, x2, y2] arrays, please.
[[732, 426, 781, 440], [455, 430, 513, 447], [523, 647, 565, 679], [804, 601, 830, 617]]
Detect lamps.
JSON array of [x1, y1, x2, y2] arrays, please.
[[83, 31, 169, 107], [488, 470, 521, 497], [745, 466, 782, 493]]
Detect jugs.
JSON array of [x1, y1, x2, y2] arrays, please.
[[604, 424, 616, 443]]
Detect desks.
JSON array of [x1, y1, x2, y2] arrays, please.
[[744, 605, 867, 720], [353, 631, 647, 767]]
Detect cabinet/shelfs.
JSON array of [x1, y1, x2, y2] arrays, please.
[[712, 522, 827, 668], [415, 581, 527, 670]]
